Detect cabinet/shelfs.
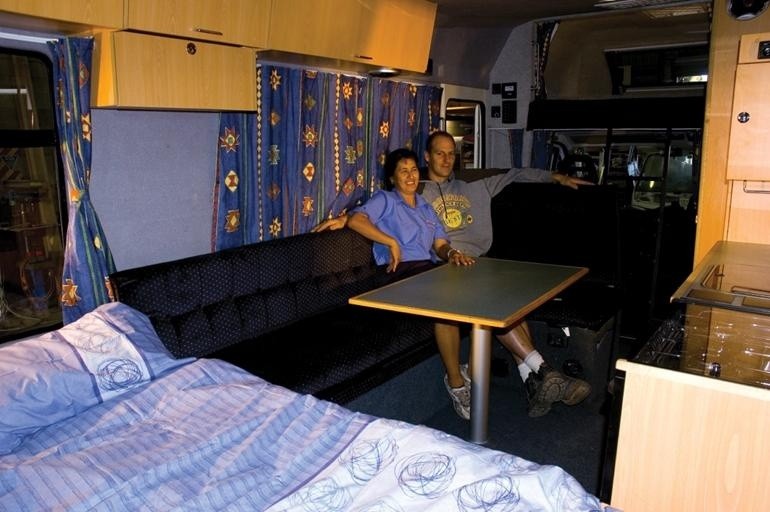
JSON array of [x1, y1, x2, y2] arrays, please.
[[725, 32, 770, 182], [89, 32, 258, 113], [124, 1, 272, 48], [1, 1, 129, 35], [260, 2, 438, 74]]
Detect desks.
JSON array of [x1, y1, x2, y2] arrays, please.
[[347, 257, 591, 446]]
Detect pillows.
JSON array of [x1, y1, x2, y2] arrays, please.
[[0, 301, 197, 454]]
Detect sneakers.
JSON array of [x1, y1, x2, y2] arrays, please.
[[443, 364, 471, 421]]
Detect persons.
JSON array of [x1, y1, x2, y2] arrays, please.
[[344, 146, 592, 420], [307, 130, 601, 419]]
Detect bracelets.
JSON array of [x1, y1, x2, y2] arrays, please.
[[447, 248, 463, 258]]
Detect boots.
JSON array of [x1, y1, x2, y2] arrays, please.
[[522, 372, 566, 419], [537, 361, 593, 407]]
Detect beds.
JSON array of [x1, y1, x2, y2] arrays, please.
[[0, 301, 622, 512]]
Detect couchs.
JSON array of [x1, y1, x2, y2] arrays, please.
[[108, 168, 629, 406]]
